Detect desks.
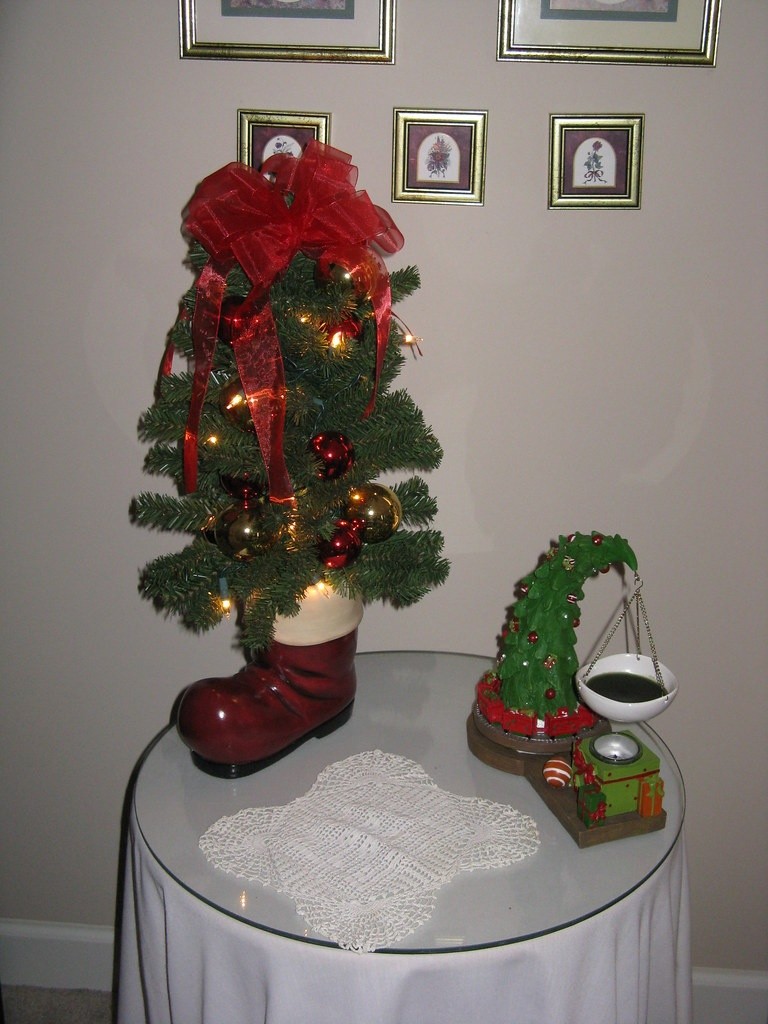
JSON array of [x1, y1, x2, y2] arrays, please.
[[117, 650, 694, 1023]]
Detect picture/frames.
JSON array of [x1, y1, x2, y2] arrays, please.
[[236, 108, 332, 180], [175, 0, 396, 65], [548, 114, 644, 210], [496, 0, 721, 68], [391, 107, 489, 208]]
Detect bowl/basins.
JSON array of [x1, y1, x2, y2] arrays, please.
[[575, 653, 679, 723]]
